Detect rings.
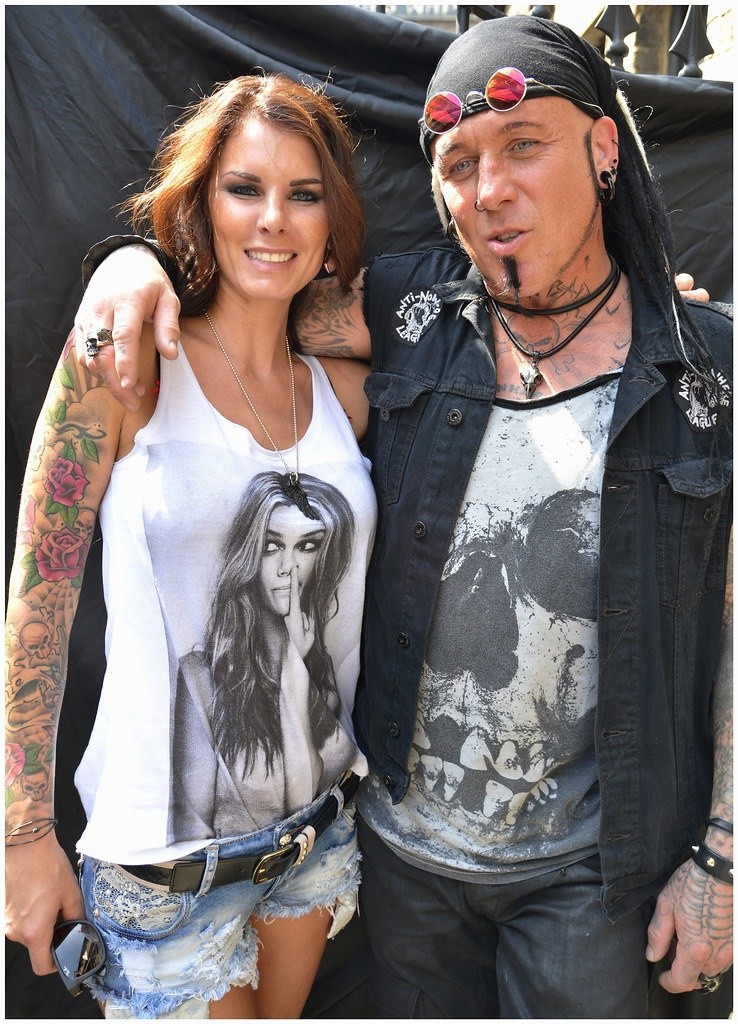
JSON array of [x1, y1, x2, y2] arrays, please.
[[86, 329, 112, 356], [699, 973, 722, 994]]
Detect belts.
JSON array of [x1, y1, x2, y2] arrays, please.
[[113, 774, 360, 894]]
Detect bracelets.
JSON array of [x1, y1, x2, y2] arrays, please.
[[706, 818, 732, 833], [5, 819, 57, 847], [689, 842, 733, 882]]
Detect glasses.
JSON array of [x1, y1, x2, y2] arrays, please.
[[50, 920, 106, 998], [418, 66, 605, 135]]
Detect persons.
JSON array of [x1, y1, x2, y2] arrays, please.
[[5, 77, 710, 1019], [73, 16, 733, 1019]]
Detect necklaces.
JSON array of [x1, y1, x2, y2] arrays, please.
[[499, 255, 614, 316], [204, 309, 319, 520], [490, 266, 620, 400]]
